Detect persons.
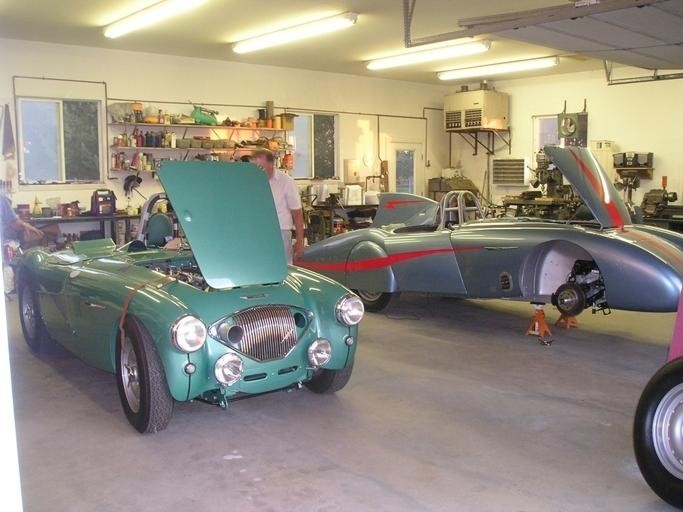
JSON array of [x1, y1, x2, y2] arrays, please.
[[250, 150, 304, 266], [1, 195, 44, 282]]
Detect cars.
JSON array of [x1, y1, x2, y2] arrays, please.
[[15, 158, 365, 432]]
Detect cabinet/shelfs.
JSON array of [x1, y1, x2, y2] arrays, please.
[[313, 204, 379, 237], [108, 122, 295, 173]]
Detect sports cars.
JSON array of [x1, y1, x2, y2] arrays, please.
[[289, 145, 683, 314]]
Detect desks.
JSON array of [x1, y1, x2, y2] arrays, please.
[[29, 213, 141, 247]]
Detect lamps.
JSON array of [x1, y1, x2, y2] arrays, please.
[[365, 39, 491, 70], [436, 56, 560, 81], [232, 12, 357, 54], [103, 0, 205, 39]]
